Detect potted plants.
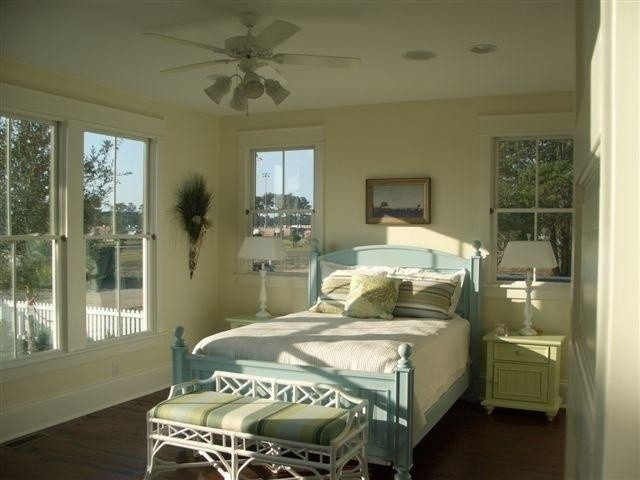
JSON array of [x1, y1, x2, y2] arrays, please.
[[167, 173, 215, 270]]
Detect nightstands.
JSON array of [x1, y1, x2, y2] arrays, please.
[[225, 314, 281, 329], [480, 331, 567, 421]]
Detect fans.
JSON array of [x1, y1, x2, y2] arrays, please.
[[140, 12, 361, 77]]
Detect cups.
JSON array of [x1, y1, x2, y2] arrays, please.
[[494, 323, 508, 338]]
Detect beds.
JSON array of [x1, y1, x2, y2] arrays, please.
[[171, 240, 484, 480]]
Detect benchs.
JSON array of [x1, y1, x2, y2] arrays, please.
[[143, 371, 370, 480]]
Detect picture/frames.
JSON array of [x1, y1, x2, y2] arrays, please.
[[365, 177, 431, 225]]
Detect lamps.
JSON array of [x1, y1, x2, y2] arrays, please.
[[237, 237, 290, 318], [205, 58, 290, 111], [499, 240, 558, 336]]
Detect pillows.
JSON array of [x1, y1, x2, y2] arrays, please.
[[308, 260, 466, 320], [341, 272, 402, 320]]
